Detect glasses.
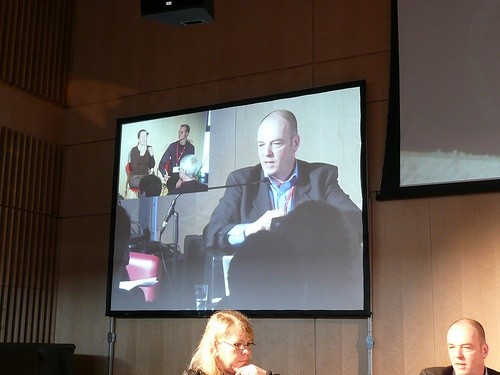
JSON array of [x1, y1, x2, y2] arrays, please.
[[215, 339, 255, 349]]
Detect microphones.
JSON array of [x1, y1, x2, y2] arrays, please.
[[173, 177, 270, 200]]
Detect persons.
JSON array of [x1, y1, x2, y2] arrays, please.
[[129, 129, 155, 189], [159, 124, 195, 193], [167, 154, 208, 195], [182, 310, 281, 375], [203, 110, 363, 254], [138, 175, 161, 197], [419, 318, 500, 375]]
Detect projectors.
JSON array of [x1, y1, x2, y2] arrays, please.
[[140, 0, 215, 26]]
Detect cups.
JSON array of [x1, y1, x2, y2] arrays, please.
[[195, 284, 208, 301]]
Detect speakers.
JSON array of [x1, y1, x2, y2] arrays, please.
[[0, 343, 75, 375]]
